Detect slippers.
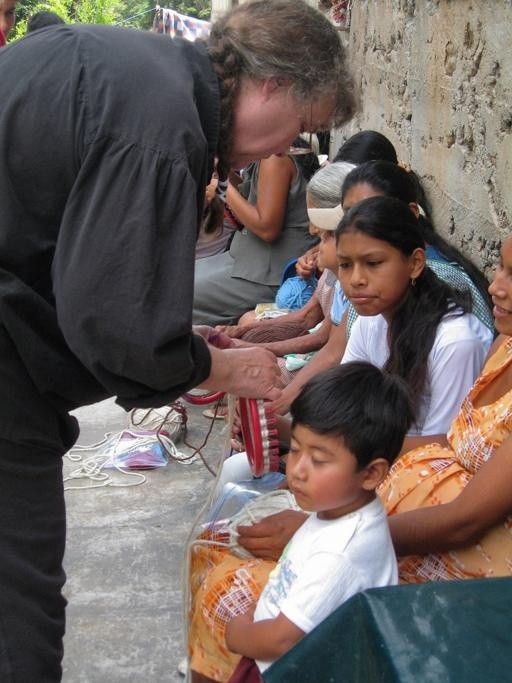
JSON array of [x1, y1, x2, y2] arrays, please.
[[202, 399, 228, 419]]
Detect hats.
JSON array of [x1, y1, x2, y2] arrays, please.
[[307, 202, 344, 231]]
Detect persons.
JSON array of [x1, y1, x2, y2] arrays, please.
[[214, 163, 358, 359], [223, 362, 418, 683], [187, 228, 512, 683], [0, 0, 16, 49], [191, 137, 321, 329], [262, 203, 344, 417], [339, 164, 497, 351], [0, 0, 363, 683], [295, 130, 411, 280]]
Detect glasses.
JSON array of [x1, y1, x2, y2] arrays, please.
[[287, 100, 316, 155]]
